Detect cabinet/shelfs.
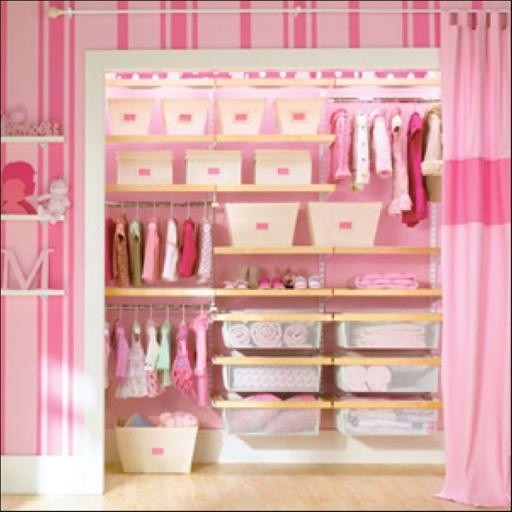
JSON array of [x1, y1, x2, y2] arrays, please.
[[102, 76, 441, 412], [0, 133, 68, 298]]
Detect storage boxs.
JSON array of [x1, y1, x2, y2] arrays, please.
[[116, 149, 175, 188], [306, 200, 382, 247], [184, 149, 245, 186], [211, 399, 331, 434], [160, 94, 211, 136], [337, 311, 441, 350], [213, 96, 268, 136], [273, 94, 324, 137], [114, 412, 201, 476], [211, 356, 339, 393], [105, 94, 155, 137], [253, 148, 313, 185], [211, 311, 337, 347], [335, 354, 439, 393], [333, 401, 439, 435], [220, 203, 302, 245]]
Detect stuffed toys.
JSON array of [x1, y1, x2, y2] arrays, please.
[[38, 177, 70, 223]]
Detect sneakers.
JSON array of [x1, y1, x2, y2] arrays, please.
[[308, 276, 321, 288], [223, 281, 234, 288], [258, 277, 271, 288], [272, 278, 284, 288], [290, 276, 307, 288], [235, 281, 249, 289]]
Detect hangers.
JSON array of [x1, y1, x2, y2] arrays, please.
[[112, 301, 211, 331], [324, 95, 439, 121]]
